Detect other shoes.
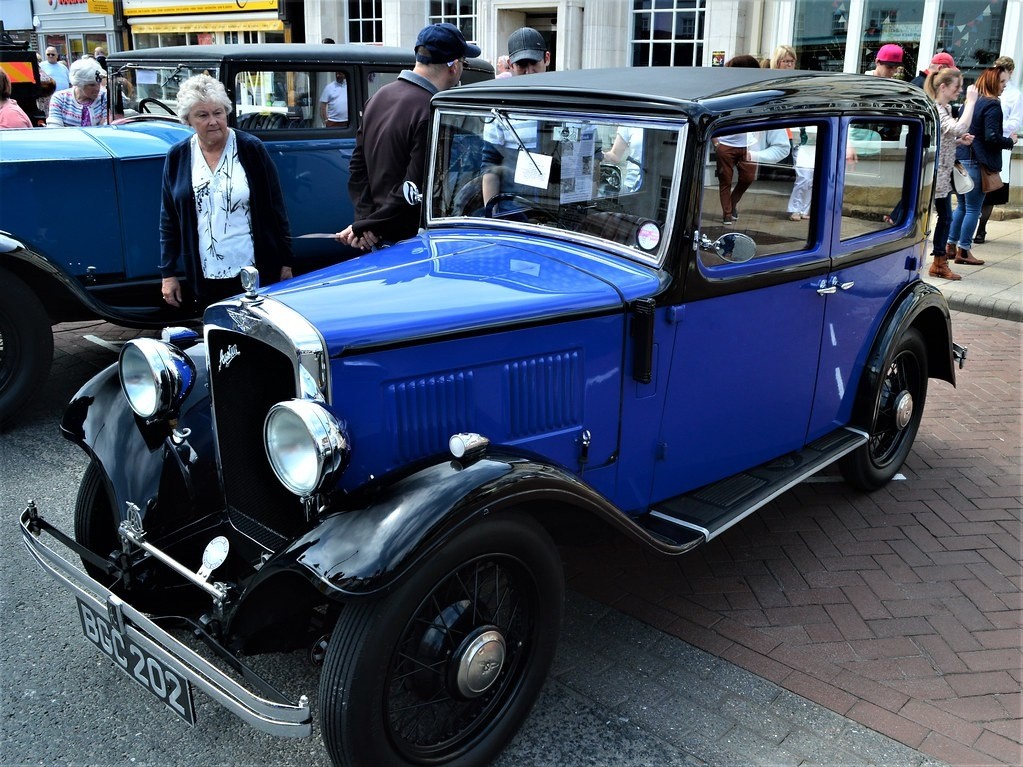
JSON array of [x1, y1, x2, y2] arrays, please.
[[724, 219, 734, 225], [802, 215, 809, 219], [732, 212, 739, 220], [790, 213, 801, 221], [974, 230, 986, 244]]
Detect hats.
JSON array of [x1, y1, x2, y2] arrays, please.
[[932, 52, 961, 72], [877, 44, 904, 62], [508, 26, 547, 65], [415, 23, 481, 65]]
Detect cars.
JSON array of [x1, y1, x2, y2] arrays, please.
[[18, 66, 969, 767], [0, 44, 497, 435]]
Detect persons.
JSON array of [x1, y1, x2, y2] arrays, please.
[[35, 46, 138, 127], [319, 71, 348, 128], [711, 46, 817, 225], [334, 23, 643, 253], [158, 74, 292, 307], [0, 66, 33, 129], [847, 44, 1018, 280]]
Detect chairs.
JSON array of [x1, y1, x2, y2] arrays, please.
[[233, 111, 313, 129]]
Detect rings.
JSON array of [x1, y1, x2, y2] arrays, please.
[[163, 297, 165, 299]]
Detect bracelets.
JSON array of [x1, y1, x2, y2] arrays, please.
[[715, 142, 718, 146]]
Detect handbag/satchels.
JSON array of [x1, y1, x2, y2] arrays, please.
[[981, 166, 1004, 193], [951, 160, 974, 194]]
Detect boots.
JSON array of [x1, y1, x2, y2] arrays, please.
[[929, 254, 961, 280], [955, 248, 985, 265], [945, 243, 957, 260]]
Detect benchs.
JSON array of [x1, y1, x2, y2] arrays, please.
[[579, 213, 659, 257]]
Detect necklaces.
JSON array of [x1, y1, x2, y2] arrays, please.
[[208, 142, 223, 166]]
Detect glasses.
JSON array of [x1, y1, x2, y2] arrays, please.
[[46, 52, 56, 56], [781, 60, 796, 64], [880, 61, 903, 68], [459, 60, 469, 68]]
